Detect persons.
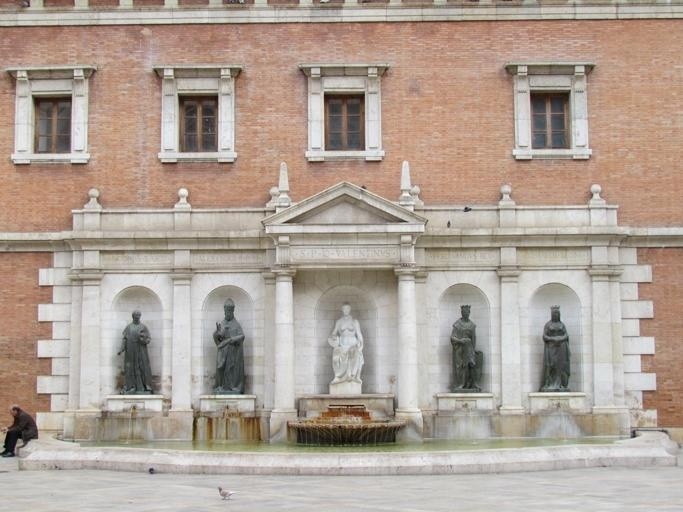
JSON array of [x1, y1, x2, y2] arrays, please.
[[213, 297, 245, 394], [117, 310, 153, 395], [330, 303, 364, 385], [0, 405, 38, 457], [450, 305, 482, 390], [543, 306, 570, 389]]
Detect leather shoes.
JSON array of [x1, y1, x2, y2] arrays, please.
[[1, 451, 14, 457]]
